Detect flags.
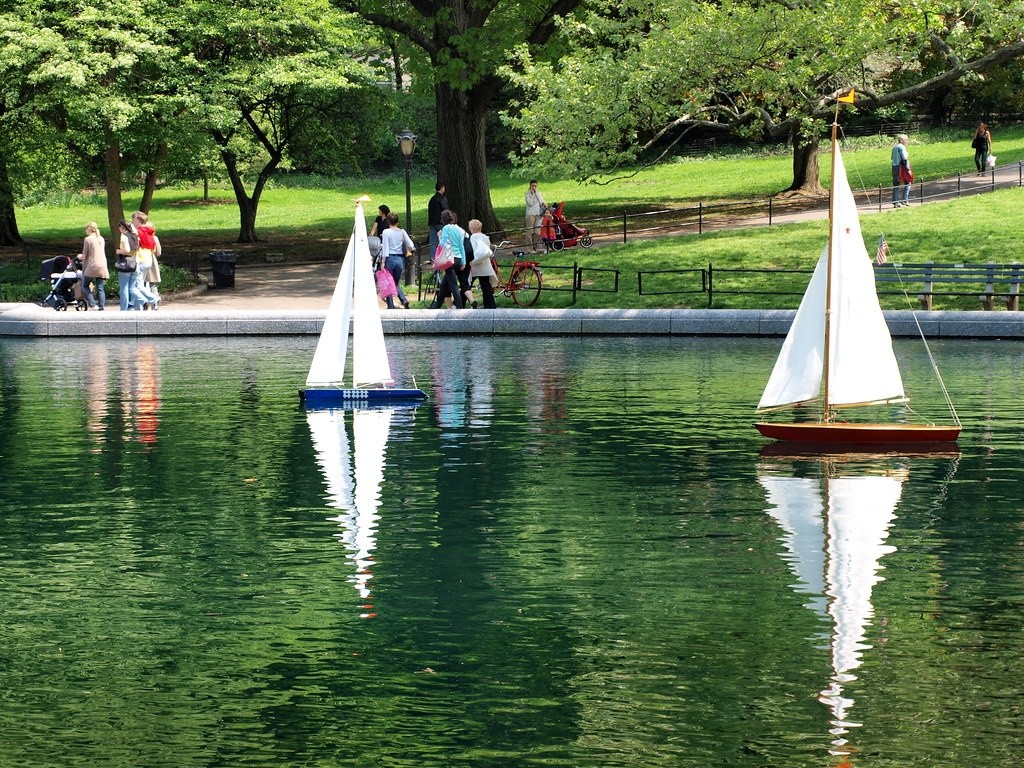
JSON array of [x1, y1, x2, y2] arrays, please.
[[877, 234, 888, 265]]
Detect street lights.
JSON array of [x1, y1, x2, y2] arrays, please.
[[397, 126, 418, 286]]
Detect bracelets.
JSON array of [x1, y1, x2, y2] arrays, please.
[[382, 261, 385, 263]]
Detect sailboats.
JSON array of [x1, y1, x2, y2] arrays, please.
[[751, 97, 966, 449], [298, 201, 432, 404]]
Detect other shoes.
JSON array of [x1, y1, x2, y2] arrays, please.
[[529, 250, 538, 253], [902, 201, 910, 206], [452, 305, 457, 309], [83, 297, 162, 310], [893, 203, 900, 208], [535, 247, 545, 252], [471, 300, 477, 310]]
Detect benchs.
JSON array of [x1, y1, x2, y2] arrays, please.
[[994, 262, 1024, 311], [870, 259, 1003, 311]]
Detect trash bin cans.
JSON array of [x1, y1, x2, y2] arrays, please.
[[210, 250, 236, 286]]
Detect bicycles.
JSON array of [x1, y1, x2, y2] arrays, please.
[[423, 240, 544, 309]]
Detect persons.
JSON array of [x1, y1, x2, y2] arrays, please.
[[381, 213, 414, 309], [77, 222, 109, 311], [432, 209, 496, 309], [525, 179, 546, 253], [561, 215, 585, 233], [538, 208, 556, 246], [370, 205, 391, 243], [428, 182, 449, 260], [974, 123, 992, 177], [891, 134, 912, 208], [116, 211, 162, 310]]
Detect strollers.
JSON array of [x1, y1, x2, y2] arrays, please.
[[41, 254, 88, 312], [547, 201, 594, 253]]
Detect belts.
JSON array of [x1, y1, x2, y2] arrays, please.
[[390, 254, 403, 257]]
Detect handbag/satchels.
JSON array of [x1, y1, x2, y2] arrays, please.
[[470, 236, 492, 264], [114, 253, 137, 272], [433, 234, 455, 269], [464, 232, 474, 262], [404, 230, 412, 257], [898, 160, 914, 183], [972, 138, 977, 148], [375, 266, 398, 299], [986, 154, 997, 166]]
